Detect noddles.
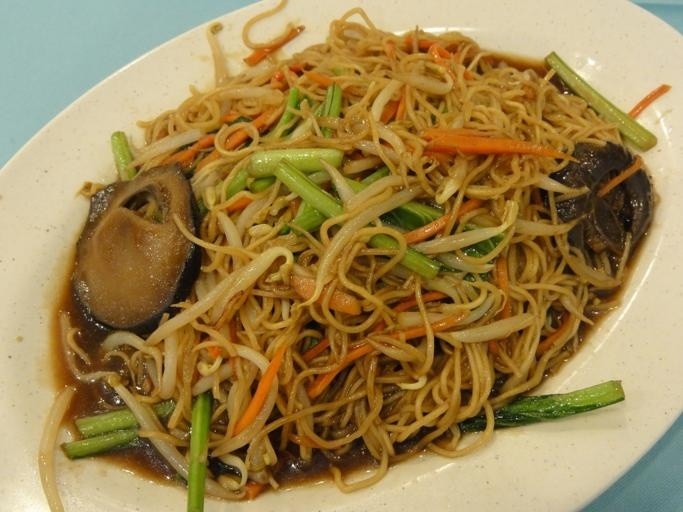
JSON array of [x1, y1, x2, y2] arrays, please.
[[130, 0, 632, 491]]
